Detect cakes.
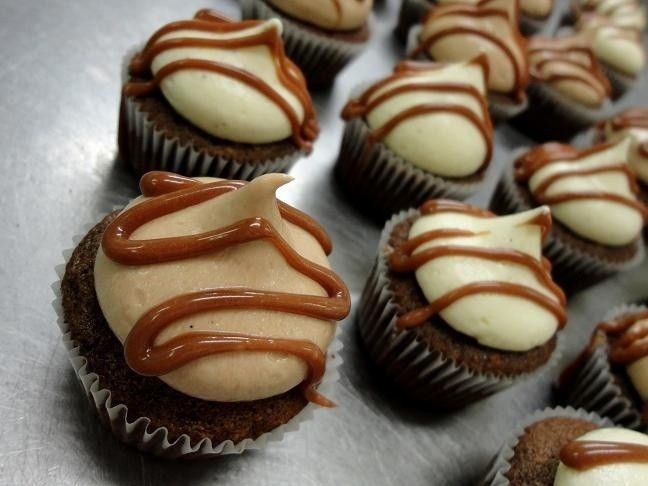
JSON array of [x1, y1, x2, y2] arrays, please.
[[49, 0, 648, 486]]
